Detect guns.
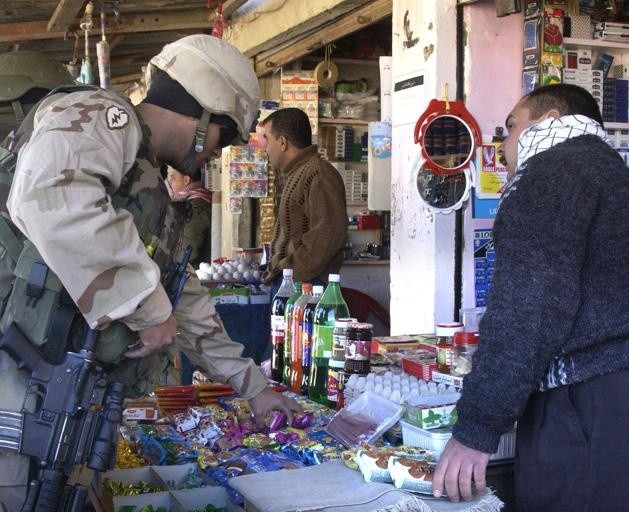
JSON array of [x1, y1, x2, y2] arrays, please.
[[0, 286, 126, 511]]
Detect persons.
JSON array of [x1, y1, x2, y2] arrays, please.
[[1, 31, 305, 509], [165, 165, 211, 273], [430, 85, 628, 512], [259, 107, 350, 364]]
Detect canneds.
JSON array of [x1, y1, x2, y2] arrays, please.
[[327, 317, 373, 410], [424, 118, 471, 156]]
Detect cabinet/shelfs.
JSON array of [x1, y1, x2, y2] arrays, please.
[[521, 0, 628, 150], [290, 56, 381, 207]]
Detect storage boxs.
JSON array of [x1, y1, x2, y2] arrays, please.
[[98, 463, 248, 512]]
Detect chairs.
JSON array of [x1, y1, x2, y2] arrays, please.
[[340, 287, 390, 336]]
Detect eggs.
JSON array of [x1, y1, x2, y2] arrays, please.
[[193, 258, 261, 283]]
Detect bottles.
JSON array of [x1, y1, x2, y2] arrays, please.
[[452, 333, 479, 378], [344, 241, 380, 260], [267, 268, 350, 404]]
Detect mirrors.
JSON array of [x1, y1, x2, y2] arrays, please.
[[414, 98, 483, 175], [410, 158, 477, 216]]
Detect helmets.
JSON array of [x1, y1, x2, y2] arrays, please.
[[0, 51, 76, 106], [143, 32, 261, 148]]
